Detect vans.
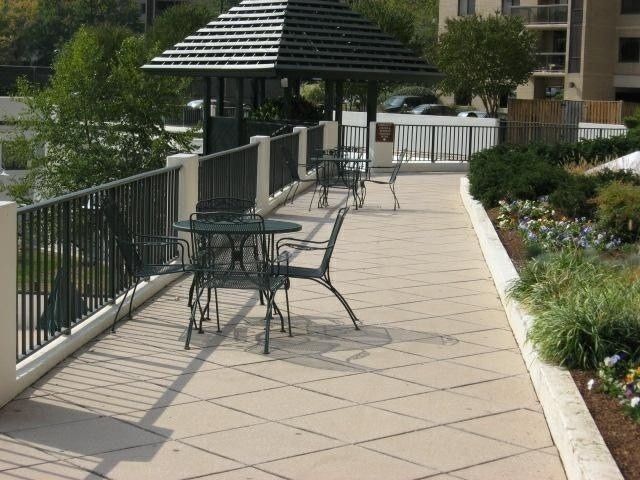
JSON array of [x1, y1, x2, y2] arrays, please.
[[379, 96, 439, 114]]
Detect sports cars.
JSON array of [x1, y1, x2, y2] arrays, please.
[[457, 111, 508, 119]]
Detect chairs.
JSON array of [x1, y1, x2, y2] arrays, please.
[[264, 207, 359, 331], [359, 148, 408, 211], [99, 198, 204, 331], [196, 197, 256, 225], [308, 150, 358, 212], [330, 146, 357, 162], [283, 160, 320, 208], [184, 212, 292, 354]]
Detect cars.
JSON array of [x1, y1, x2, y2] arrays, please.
[[400, 102, 459, 116], [187, 99, 233, 108]]
